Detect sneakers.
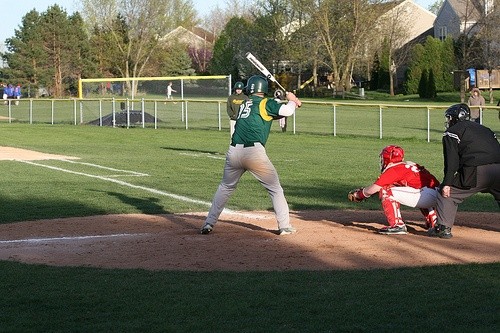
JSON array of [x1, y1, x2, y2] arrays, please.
[[428, 224, 453, 238], [378, 224, 407, 235], [200, 222, 213, 233], [279, 227, 297, 235]]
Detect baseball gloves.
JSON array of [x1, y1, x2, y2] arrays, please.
[[347, 188, 362, 204]]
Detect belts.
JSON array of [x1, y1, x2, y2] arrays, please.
[[231, 142, 264, 148]]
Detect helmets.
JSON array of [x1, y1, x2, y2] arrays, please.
[[234, 81, 244, 91], [445, 103, 471, 124], [244, 75, 268, 96], [378, 145, 404, 170]]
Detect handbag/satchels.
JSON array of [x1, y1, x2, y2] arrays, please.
[[452, 166, 477, 189]]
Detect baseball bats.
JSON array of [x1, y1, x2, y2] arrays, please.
[[244, 51, 302, 109]]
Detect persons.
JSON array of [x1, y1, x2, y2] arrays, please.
[[163, 81, 178, 104], [467, 87, 485, 124], [200, 74, 301, 235], [497, 99, 500, 119], [425, 102, 500, 239], [85, 81, 124, 96], [279, 92, 287, 131], [226, 81, 250, 145], [2, 82, 21, 105], [348, 144, 442, 235]]
